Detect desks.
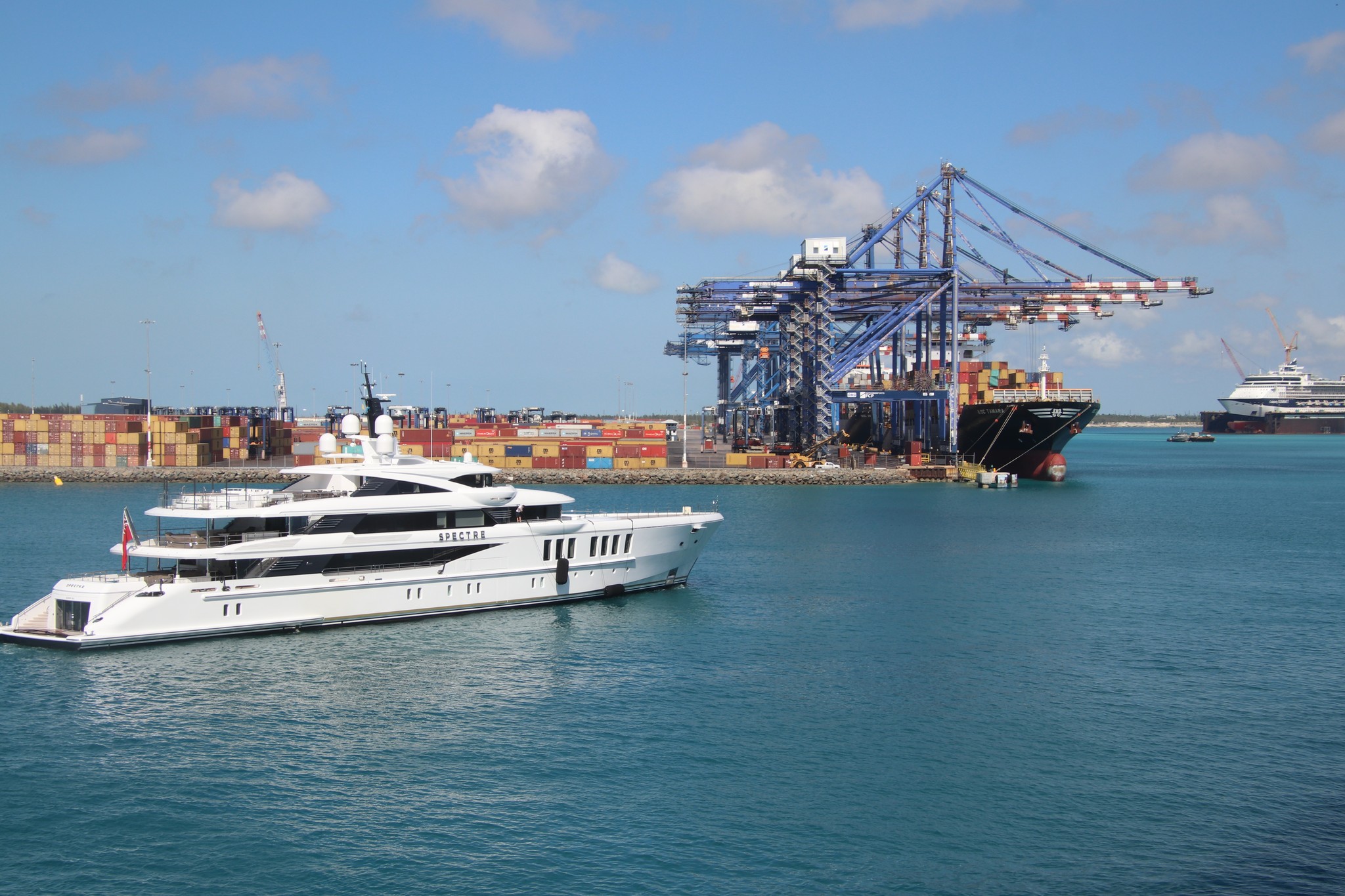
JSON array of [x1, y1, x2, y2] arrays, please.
[[165, 528, 228, 547]]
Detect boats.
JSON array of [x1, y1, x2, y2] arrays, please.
[[0, 357, 725, 653], [1190, 434, 1215, 442], [1166, 426, 1190, 442], [836, 323, 1101, 481], [1217, 358, 1345, 421]]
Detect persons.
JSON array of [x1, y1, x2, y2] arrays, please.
[[515, 505, 525, 523]]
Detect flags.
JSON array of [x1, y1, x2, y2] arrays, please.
[[120, 509, 134, 572]]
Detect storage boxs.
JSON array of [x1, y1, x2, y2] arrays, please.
[[724, 357, 1064, 469], [0, 408, 672, 471], [677, 423, 703, 430]]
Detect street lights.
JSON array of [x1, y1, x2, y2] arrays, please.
[[139, 317, 157, 467], [398, 372, 405, 406], [272, 342, 282, 420], [419, 380, 424, 407], [350, 364, 359, 415], [446, 383, 451, 429]]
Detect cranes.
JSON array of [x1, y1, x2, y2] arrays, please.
[[1220, 338, 1246, 381], [1265, 308, 1299, 362], [254, 310, 279, 404], [663, 162, 1216, 467]]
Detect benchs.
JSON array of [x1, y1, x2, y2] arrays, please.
[[272, 489, 347, 499], [136, 570, 173, 584]]
